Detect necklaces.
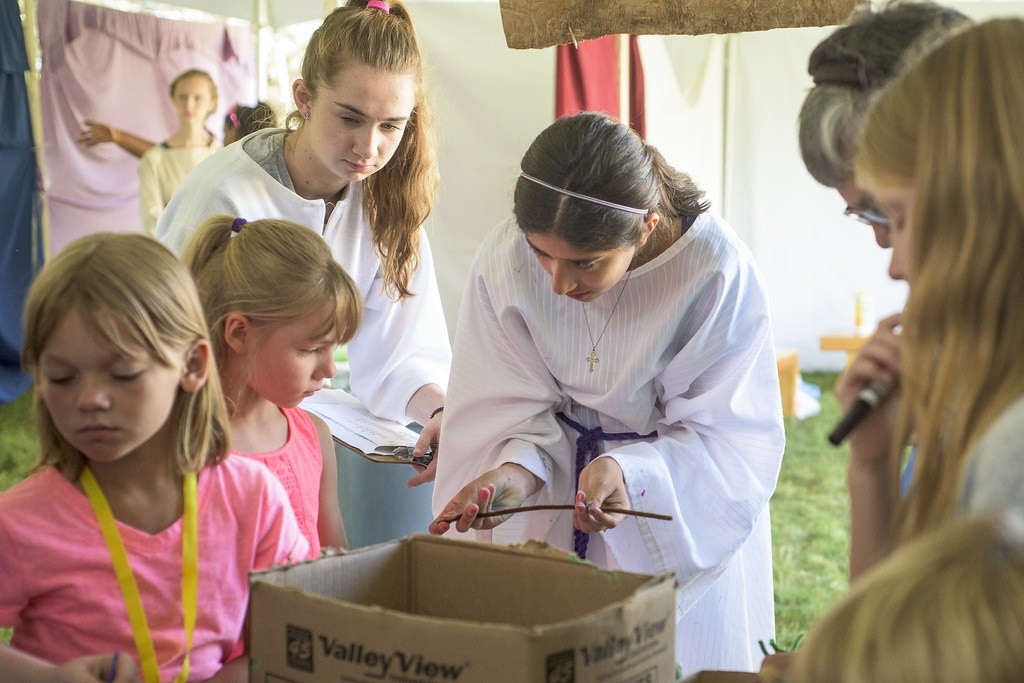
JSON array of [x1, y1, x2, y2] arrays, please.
[[576, 247, 639, 374]]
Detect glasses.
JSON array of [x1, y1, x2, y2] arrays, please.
[[844, 202, 889, 226]]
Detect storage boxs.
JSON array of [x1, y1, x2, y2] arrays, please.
[[248, 532, 679, 683]]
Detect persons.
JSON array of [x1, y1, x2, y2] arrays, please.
[[0, 231, 309, 683], [685, 0, 1024, 683], [181, 212, 362, 567], [431, 113, 786, 676], [78, 0, 453, 489]]
